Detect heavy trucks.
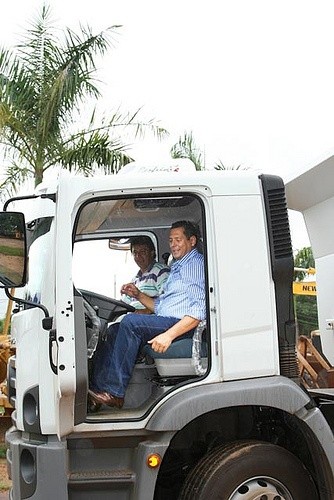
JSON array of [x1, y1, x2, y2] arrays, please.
[[0, 146, 334, 500]]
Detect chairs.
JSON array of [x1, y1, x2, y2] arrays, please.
[[146, 320, 209, 378]]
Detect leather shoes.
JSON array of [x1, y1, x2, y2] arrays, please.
[[87, 389, 124, 412]]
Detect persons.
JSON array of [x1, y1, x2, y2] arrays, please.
[[86, 220, 206, 413], [119, 234, 170, 315]]
